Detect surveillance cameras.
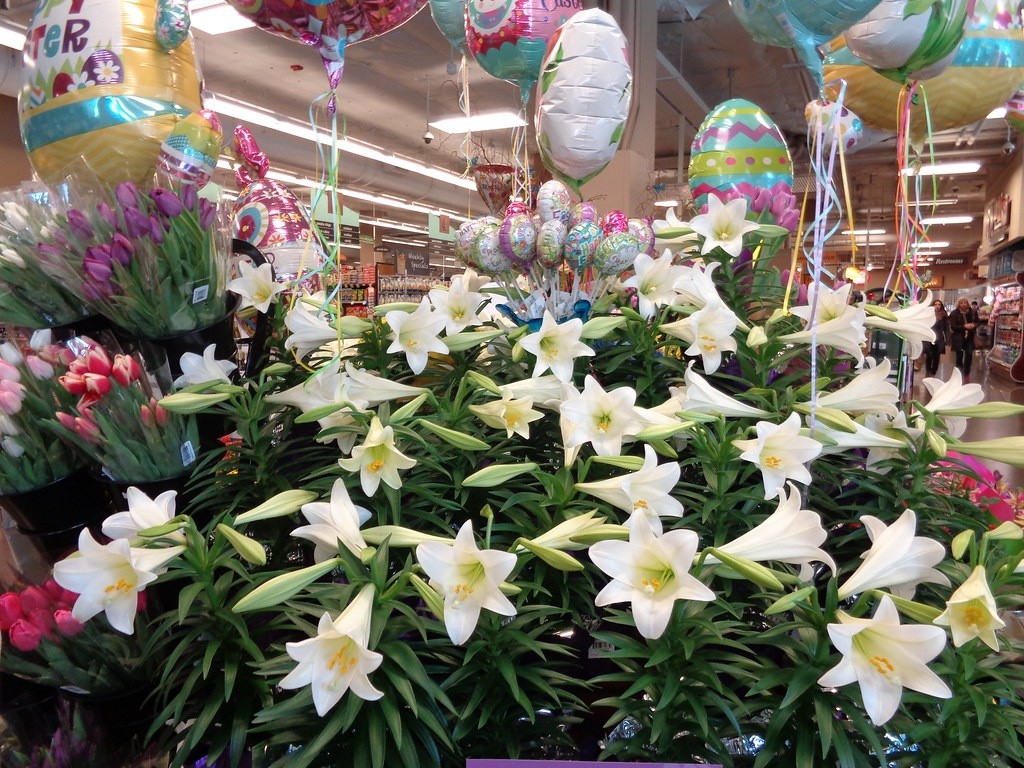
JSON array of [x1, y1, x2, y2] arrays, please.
[[1003, 142, 1015, 155], [952, 186, 960, 193], [423, 131, 434, 145]]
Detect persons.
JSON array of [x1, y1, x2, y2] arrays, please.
[[949, 298, 981, 383], [923, 300, 951, 378]]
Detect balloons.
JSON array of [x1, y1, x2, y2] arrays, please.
[[464, 0, 583, 110], [533, 7, 633, 198], [687, 98, 794, 254], [224, 127, 322, 338], [729, 0, 1024, 166], [226, 0, 431, 113], [17, 0, 224, 198], [429, 0, 468, 55], [455, 179, 656, 277]]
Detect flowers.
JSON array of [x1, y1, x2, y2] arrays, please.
[[36, 337, 200, 480], [50, 193, 1024, 768], [0, 328, 88, 494], [32, 171, 227, 336], [10, 700, 97, 768], [0, 575, 147, 696], [1, 196, 93, 327]]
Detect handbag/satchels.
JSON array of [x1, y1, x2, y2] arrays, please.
[[951, 333, 964, 352]]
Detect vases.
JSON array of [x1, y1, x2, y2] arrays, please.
[[31, 305, 133, 356], [72, 684, 166, 747], [121, 290, 243, 385], [97, 447, 200, 509], [1, 462, 128, 568]]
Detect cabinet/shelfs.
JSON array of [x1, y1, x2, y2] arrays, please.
[[986, 272, 1024, 382], [326, 262, 465, 320]]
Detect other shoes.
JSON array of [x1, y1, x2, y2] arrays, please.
[[964, 374, 969, 383]]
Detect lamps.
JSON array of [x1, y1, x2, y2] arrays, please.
[[328, 242, 361, 250], [842, 228, 886, 235], [895, 199, 958, 207], [203, 88, 478, 192], [188, 0, 256, 35], [224, 190, 430, 235], [0, 18, 27, 51], [898, 161, 982, 177], [215, 156, 478, 222], [845, 268, 866, 284], [902, 241, 949, 267], [381, 235, 428, 248], [427, 108, 529, 133], [919, 214, 973, 224]]
